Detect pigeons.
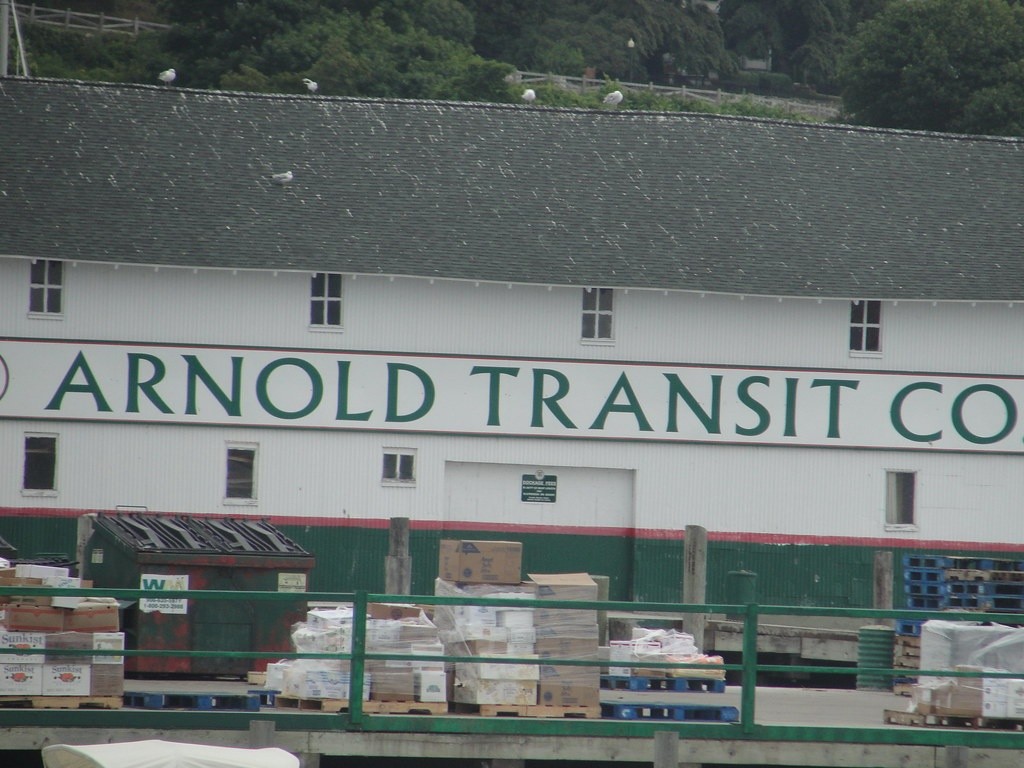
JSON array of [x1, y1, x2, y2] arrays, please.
[[603, 91, 623, 109], [521, 89, 535, 105], [157, 68, 176, 87], [268, 171, 293, 185], [302, 78, 318, 92]]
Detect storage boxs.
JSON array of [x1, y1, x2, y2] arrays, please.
[[265, 540, 724, 706], [1, 564, 126, 696], [913, 620, 1024, 719]]
